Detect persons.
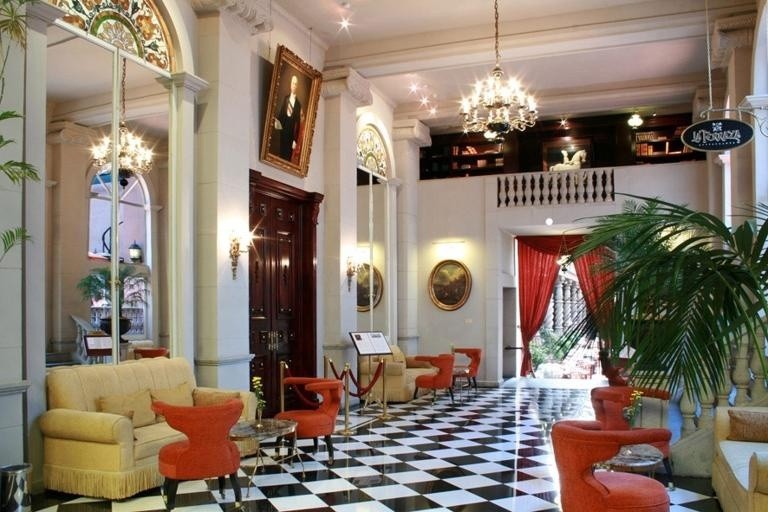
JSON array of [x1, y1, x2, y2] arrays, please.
[[273, 76, 306, 162]]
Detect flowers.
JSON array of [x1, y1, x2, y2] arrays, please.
[[623, 389, 644, 427], [252, 376, 266, 419]]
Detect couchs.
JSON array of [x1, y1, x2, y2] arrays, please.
[[711, 405, 767, 512], [37, 356, 263, 502], [358, 346, 440, 403]]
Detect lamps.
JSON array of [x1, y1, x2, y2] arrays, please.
[[627, 112, 644, 130], [459, 0, 540, 142], [128, 241, 142, 262], [89, 59, 154, 188]]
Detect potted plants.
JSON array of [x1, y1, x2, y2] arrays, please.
[[79, 265, 150, 338]]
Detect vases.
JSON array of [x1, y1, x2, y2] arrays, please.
[[253, 406, 266, 432]]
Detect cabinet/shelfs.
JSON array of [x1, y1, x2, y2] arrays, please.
[[417, 110, 707, 180]]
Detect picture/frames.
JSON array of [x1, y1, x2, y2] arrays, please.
[[257, 43, 324, 179]]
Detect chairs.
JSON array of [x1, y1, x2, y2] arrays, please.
[[152, 397, 244, 510], [274, 376, 342, 466], [413, 352, 455, 404], [553, 419, 673, 512], [452, 347, 481, 392], [591, 386, 672, 430]]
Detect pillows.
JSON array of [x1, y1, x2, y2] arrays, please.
[[150, 385, 195, 423], [98, 389, 156, 429]]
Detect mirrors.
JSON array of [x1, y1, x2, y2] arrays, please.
[[357, 166, 387, 340], [48, 24, 172, 362]]
